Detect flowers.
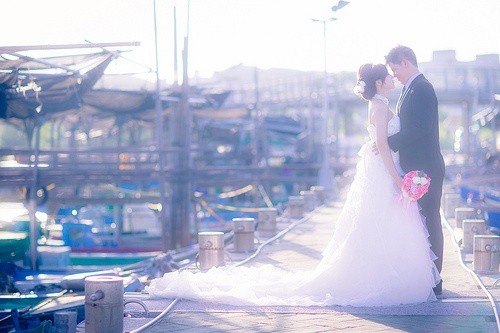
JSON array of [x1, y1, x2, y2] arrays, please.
[[394, 170, 431, 208]]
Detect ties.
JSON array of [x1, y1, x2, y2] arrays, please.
[[399, 86, 406, 110]]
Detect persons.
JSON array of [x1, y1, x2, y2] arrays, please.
[[372, 46, 445, 297], [347, 63, 441, 305]]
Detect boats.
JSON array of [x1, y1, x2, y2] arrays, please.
[[0, 47, 314, 325], [444, 95, 500, 232]]
